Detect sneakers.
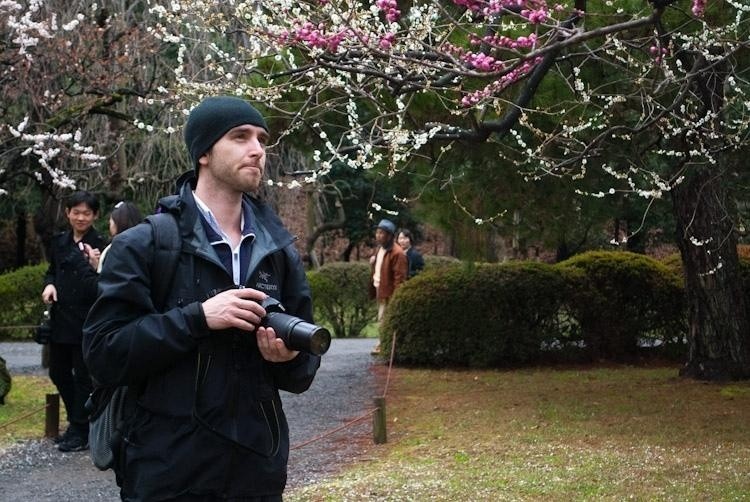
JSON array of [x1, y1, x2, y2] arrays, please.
[[59, 426, 88, 453], [55, 425, 78, 444]]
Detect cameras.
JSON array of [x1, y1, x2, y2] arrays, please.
[[250, 297, 332, 357]]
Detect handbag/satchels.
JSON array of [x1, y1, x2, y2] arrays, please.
[[33, 301, 54, 344]]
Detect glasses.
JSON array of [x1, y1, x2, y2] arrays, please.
[[115, 201, 123, 213]]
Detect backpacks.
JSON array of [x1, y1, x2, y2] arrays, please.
[[84, 213, 301, 472]]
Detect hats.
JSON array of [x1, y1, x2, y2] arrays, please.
[[184, 94, 268, 170], [371, 219, 396, 236]]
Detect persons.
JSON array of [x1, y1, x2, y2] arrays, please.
[[395, 227, 423, 281], [40, 190, 105, 453], [368, 218, 408, 355], [80, 95, 324, 500], [82, 200, 142, 306]]
